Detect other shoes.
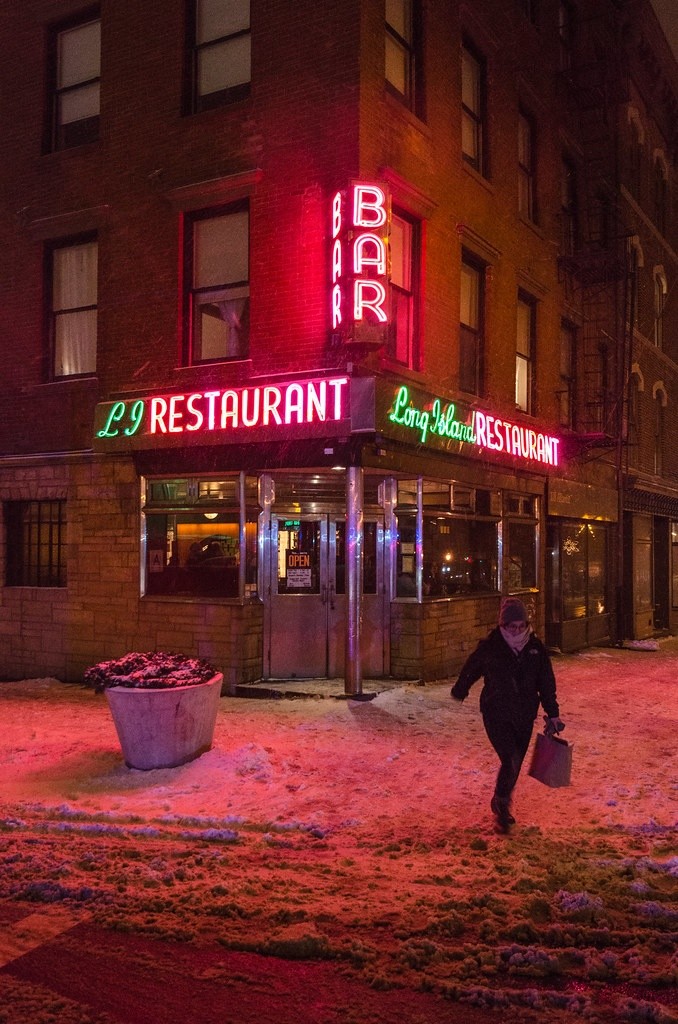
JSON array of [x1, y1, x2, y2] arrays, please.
[[491, 796, 516, 824], [493, 822, 506, 833]]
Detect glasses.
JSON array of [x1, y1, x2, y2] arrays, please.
[[505, 623, 527, 632]]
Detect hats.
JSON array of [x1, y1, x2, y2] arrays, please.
[[190, 541, 203, 552], [499, 598, 528, 624]]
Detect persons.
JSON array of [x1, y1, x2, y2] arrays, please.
[[452, 598, 564, 831]]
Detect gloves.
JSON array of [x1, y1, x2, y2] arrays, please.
[[451, 684, 469, 702], [543, 715, 565, 736]]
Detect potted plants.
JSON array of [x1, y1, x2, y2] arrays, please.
[[94, 651, 224, 771]]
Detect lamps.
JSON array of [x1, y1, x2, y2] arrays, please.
[[147, 167, 168, 185], [16, 205, 34, 224]]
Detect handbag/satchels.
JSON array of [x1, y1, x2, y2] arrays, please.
[[526, 733, 574, 788]]
